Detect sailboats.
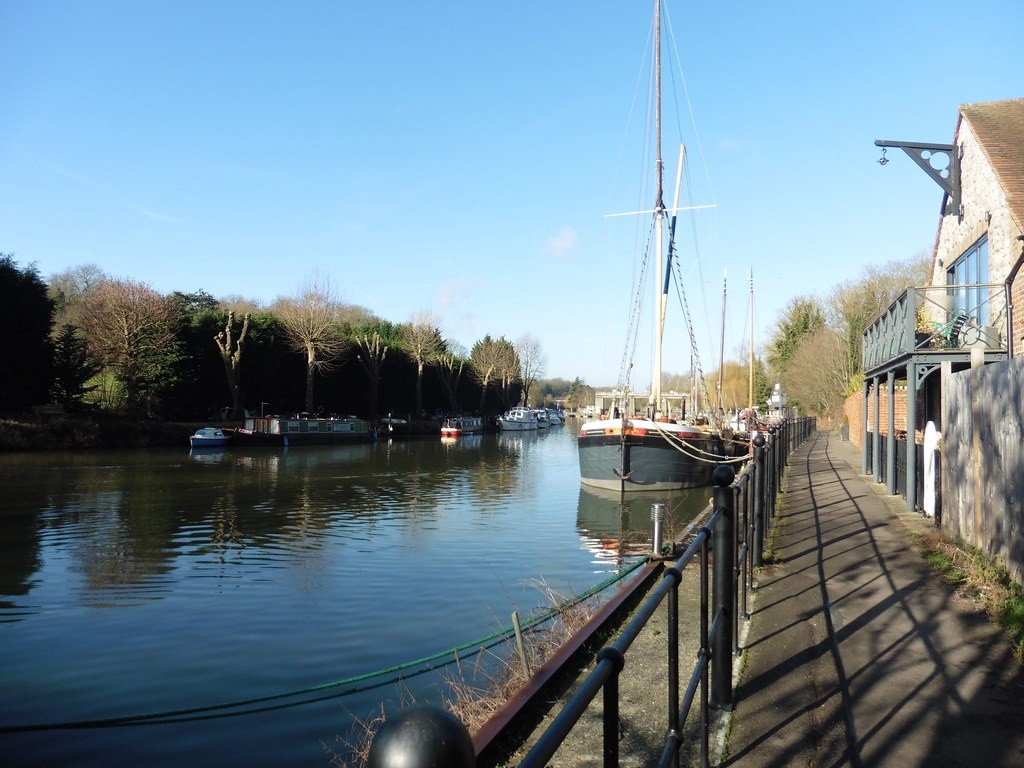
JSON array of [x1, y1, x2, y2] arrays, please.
[[575, 1, 788, 490]]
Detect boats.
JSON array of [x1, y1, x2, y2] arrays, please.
[[189, 427, 233, 447], [223, 401, 388, 441], [441, 416, 483, 437], [495, 407, 538, 430], [530, 407, 565, 429]]
